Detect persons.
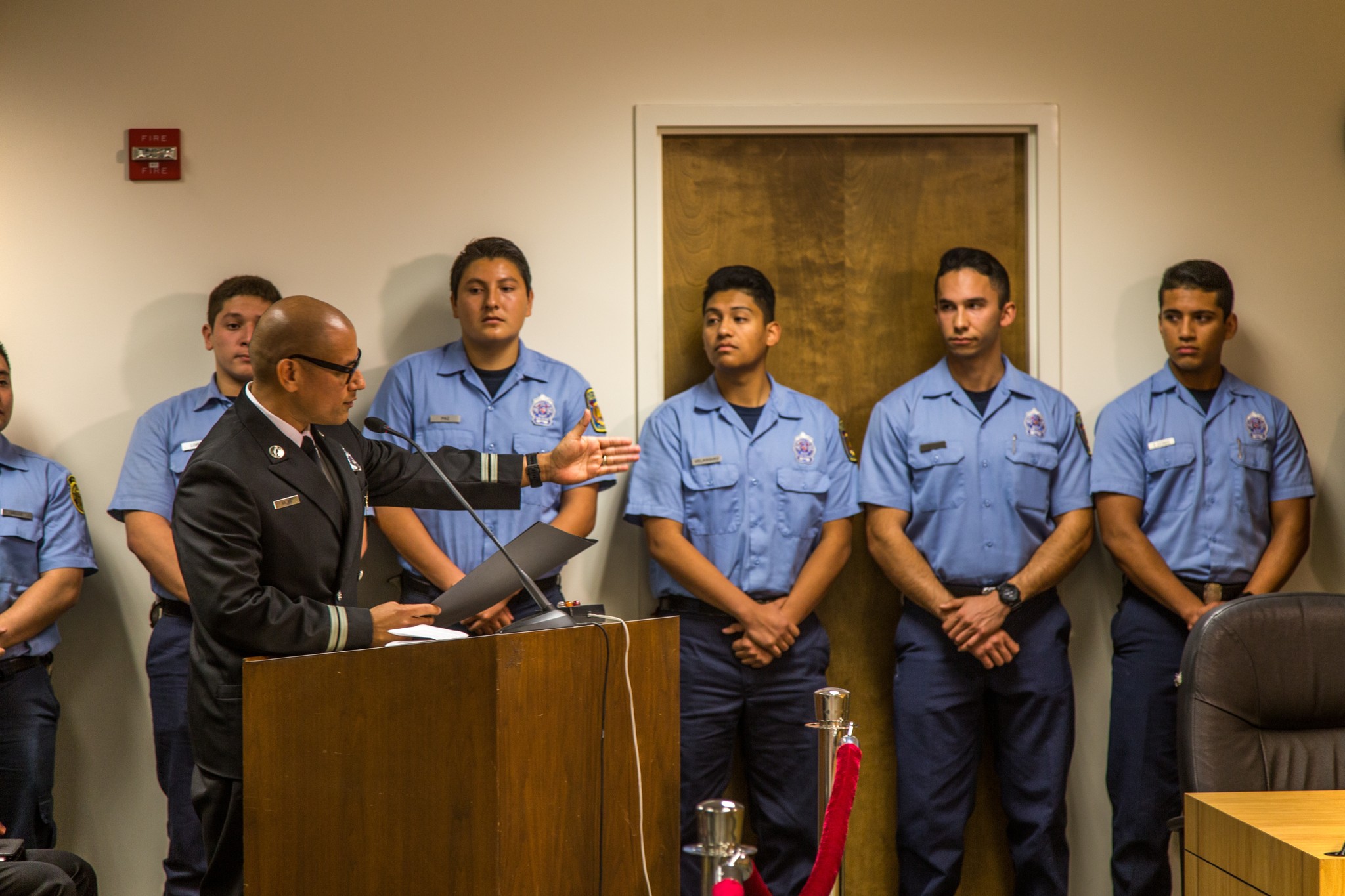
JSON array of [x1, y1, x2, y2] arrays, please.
[[170, 296, 646, 896], [1089, 259, 1317, 896], [361, 235, 618, 639], [0, 848, 99, 896], [0, 342, 99, 861], [856, 247, 1095, 896], [105, 275, 284, 896], [618, 263, 865, 895]]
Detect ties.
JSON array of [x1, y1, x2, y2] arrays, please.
[[300, 435, 322, 472]]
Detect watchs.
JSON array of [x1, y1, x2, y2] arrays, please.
[[994, 581, 1022, 612]]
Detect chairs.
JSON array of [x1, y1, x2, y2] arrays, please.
[[1163, 588, 1345, 896]]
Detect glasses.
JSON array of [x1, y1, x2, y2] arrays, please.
[[286, 347, 361, 384]]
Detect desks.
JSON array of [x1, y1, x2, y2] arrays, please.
[[1181, 787, 1345, 896]]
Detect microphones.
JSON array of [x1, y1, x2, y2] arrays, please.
[[364, 416, 607, 633]]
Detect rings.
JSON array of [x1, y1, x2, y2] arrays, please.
[[602, 455, 607, 465]]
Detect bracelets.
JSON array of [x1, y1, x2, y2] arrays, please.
[[525, 451, 543, 488]]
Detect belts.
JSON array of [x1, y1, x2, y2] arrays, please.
[[0, 651, 54, 680], [398, 570, 559, 603], [657, 589, 790, 618], [901, 578, 996, 608], [157, 596, 192, 620], [1123, 569, 1250, 602]]
